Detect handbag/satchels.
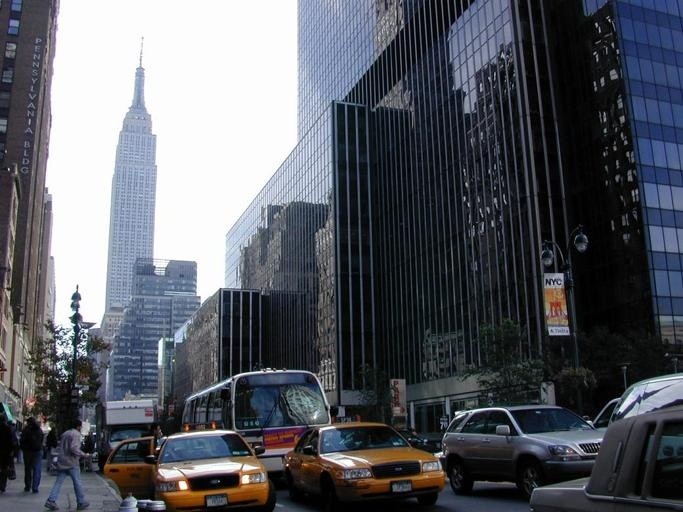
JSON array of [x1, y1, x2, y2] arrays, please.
[[7, 456, 16, 480], [57, 455, 74, 471]]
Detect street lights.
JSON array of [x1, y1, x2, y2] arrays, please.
[[616, 361, 632, 392], [540, 224, 589, 414], [70, 283, 96, 427]]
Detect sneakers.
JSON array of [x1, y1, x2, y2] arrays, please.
[[32, 488, 39, 493], [44, 497, 60, 510], [24, 487, 30, 492], [77, 501, 89, 510]]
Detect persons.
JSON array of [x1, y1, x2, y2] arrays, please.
[[137, 423, 160, 464], [0, 411, 96, 510]]
